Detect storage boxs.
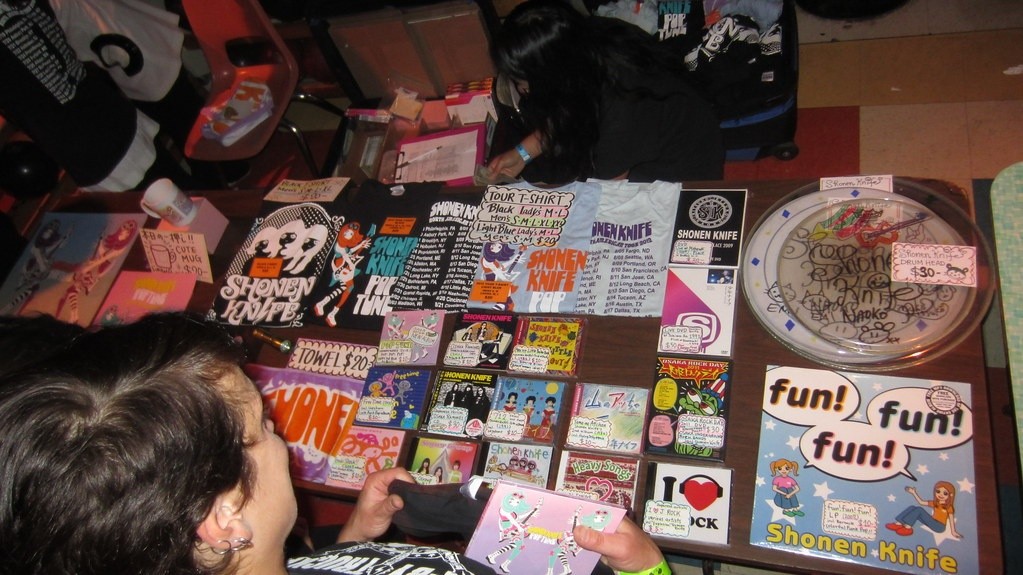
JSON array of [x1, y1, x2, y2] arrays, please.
[[157, 197, 229, 254], [342, 77, 499, 186]]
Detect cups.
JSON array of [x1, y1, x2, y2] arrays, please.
[[141, 178, 197, 227]]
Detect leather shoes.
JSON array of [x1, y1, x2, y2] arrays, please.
[[774, 142, 798, 161]]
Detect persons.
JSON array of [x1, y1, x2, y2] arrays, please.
[[1, 311, 673, 575], [486, 0, 724, 185]]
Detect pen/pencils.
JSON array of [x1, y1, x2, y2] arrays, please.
[[395, 146, 443, 168], [464, 121, 486, 125]]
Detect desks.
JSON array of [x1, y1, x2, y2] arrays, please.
[[1, 177, 1007, 575]]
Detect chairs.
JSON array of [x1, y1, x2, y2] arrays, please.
[[181, 0, 347, 181]]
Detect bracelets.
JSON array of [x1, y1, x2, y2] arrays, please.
[[514, 144, 534, 170], [612, 557, 672, 575]]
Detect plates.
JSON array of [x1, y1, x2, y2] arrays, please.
[[743, 184, 969, 361]]
